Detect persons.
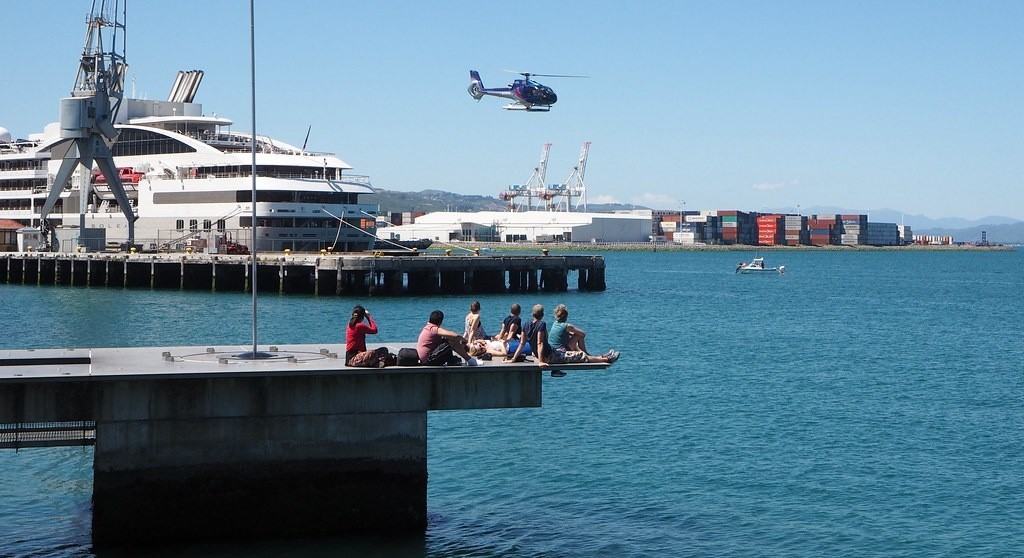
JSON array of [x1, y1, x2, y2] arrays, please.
[[495, 304, 568, 377], [462, 301, 494, 346], [548, 304, 590, 356], [505, 303, 620, 368], [344, 304, 397, 368], [417, 310, 484, 366], [760, 261, 765, 269], [736, 261, 746, 266], [126, 239, 131, 254]]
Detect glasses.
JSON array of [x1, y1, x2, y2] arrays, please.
[[479, 344, 483, 351]]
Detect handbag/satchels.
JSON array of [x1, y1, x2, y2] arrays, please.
[[385, 353, 397, 366], [504, 354, 534, 363]]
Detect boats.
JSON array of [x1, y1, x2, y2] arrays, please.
[[738, 257, 785, 273]]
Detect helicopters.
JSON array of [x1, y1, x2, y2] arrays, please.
[[465, 67, 592, 112]]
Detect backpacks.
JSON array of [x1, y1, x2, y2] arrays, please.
[[397, 348, 422, 366]]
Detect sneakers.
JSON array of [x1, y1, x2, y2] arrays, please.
[[551, 371, 566, 377], [602, 349, 620, 363]]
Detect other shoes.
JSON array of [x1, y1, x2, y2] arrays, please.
[[447, 356, 462, 366], [373, 361, 385, 368]]
